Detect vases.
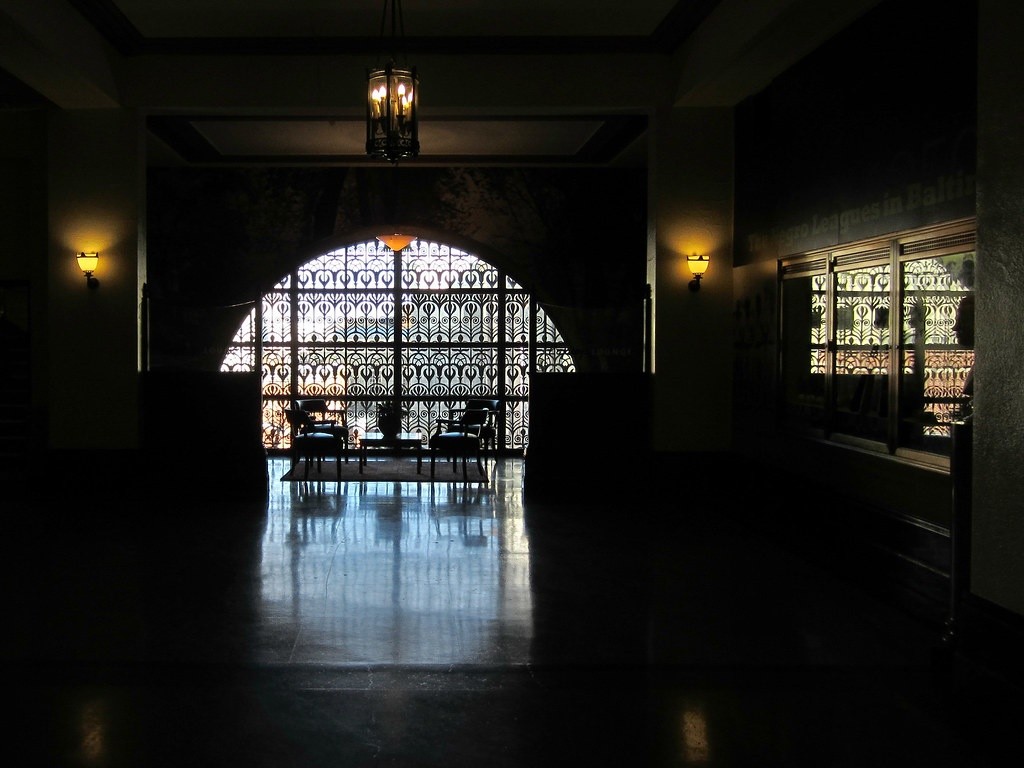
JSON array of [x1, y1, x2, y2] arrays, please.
[[379, 414, 402, 440]]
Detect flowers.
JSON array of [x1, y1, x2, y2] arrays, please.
[[376, 401, 409, 417]]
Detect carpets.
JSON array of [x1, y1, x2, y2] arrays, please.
[[281, 454, 489, 482]]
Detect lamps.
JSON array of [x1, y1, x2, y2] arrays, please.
[[365, 0, 422, 163], [77, 251, 102, 287], [376, 234, 417, 253], [686, 256, 710, 290]]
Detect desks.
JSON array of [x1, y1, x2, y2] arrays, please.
[[359, 432, 423, 474]]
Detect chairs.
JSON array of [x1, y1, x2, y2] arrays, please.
[[429, 410, 489, 482], [446, 400, 500, 461], [285, 409, 341, 473], [291, 400, 349, 461]]
